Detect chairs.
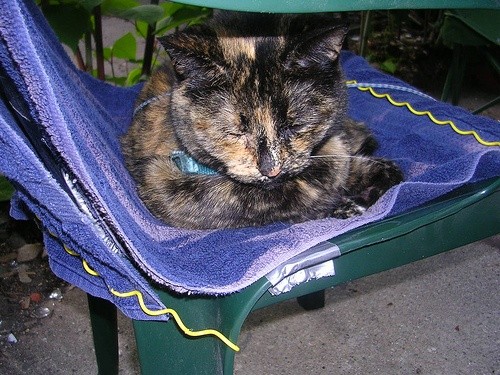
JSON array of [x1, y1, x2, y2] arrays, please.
[[0, 0, 497, 373]]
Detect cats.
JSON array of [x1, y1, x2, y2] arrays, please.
[[120, 10, 405, 230]]
